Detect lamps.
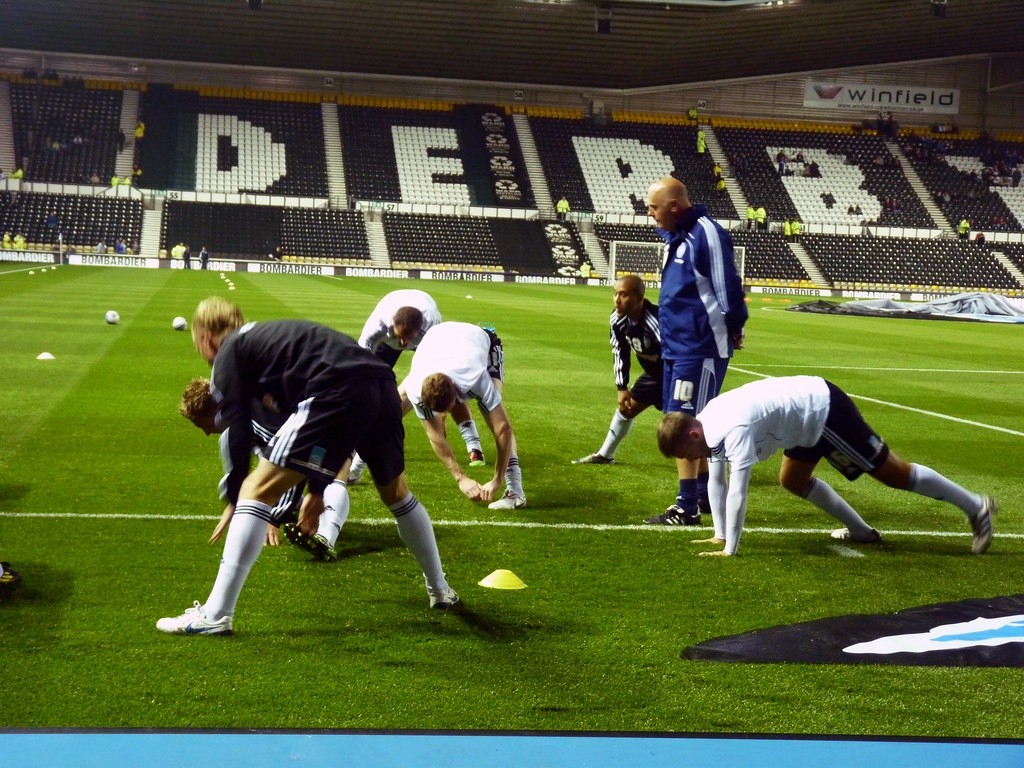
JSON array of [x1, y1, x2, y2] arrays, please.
[[930, 0, 947, 17], [246, 0, 264, 11], [595, 7, 612, 33]]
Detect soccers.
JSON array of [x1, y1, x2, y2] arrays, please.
[[173, 317, 187, 331], [107, 311, 120, 324]]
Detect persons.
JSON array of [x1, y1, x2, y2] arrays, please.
[[556, 196, 571, 221], [179, 376, 357, 562], [348, 321, 527, 509], [642, 178, 748, 526], [571, 275, 663, 464], [155, 296, 460, 634], [358, 289, 487, 466], [656, 375, 996, 557], [689, 107, 1022, 246], [0, 120, 291, 270]]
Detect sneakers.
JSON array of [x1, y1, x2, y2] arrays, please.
[[642, 504, 702, 526], [488, 489, 526, 510], [831, 527, 883, 544], [571, 454, 615, 465], [698, 499, 712, 512], [157, 601, 234, 637], [284, 523, 337, 561], [970, 494, 995, 553], [468, 449, 485, 467], [427, 586, 461, 610]]
